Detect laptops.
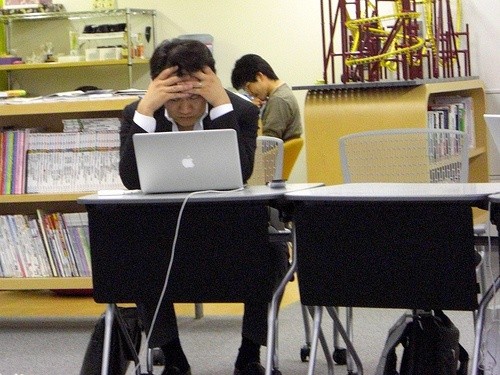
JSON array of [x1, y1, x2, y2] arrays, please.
[[483, 114, 500, 151], [133, 129, 244, 194]]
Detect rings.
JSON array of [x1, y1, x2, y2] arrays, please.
[[199, 82, 202, 87]]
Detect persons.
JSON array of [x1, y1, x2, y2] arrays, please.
[[230, 54, 302, 151], [120, 38, 289, 375]]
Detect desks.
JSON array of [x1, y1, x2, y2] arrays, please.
[[75, 183, 500, 375]]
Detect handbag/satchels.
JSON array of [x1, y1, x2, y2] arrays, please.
[[376, 306, 469, 375], [80, 305, 142, 375]]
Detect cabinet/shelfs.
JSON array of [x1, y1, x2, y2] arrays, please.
[[0, 6, 157, 88], [290, 75, 490, 230], [0, 95, 140, 290]]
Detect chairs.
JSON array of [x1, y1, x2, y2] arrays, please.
[[195, 112, 470, 375]]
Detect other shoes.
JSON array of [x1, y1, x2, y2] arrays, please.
[[234, 362, 265, 375], [161, 364, 191, 375]]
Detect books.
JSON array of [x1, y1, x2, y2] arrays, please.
[[427, 96, 465, 183], [0, 118, 124, 195], [0, 210, 92, 277]]
